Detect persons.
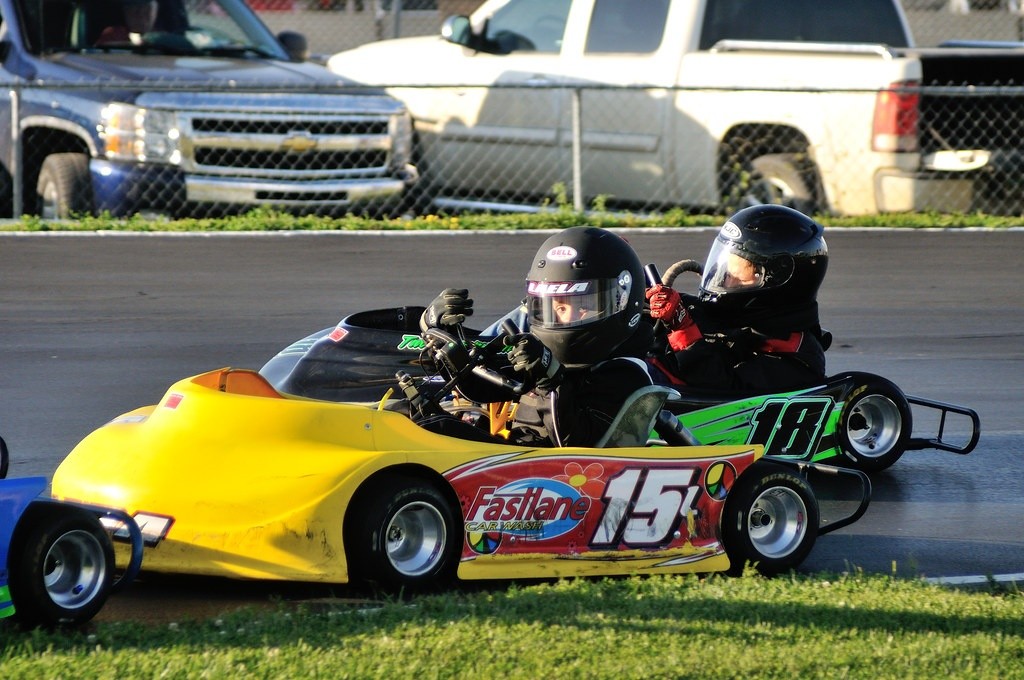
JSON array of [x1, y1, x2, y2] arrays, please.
[[478, 205, 829, 402], [418, 204, 653, 448]]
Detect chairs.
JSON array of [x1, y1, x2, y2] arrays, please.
[[596, 384, 683, 450]]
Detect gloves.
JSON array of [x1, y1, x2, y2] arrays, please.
[[419, 288, 474, 339], [646, 283, 702, 352], [502, 333, 567, 396]]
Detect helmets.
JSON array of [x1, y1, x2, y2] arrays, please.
[[698, 203, 828, 325], [525, 227, 646, 365]]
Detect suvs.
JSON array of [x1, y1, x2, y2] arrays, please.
[[1, 0, 421, 221]]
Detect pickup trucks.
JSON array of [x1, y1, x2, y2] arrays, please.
[[324, 1, 1024, 220]]
